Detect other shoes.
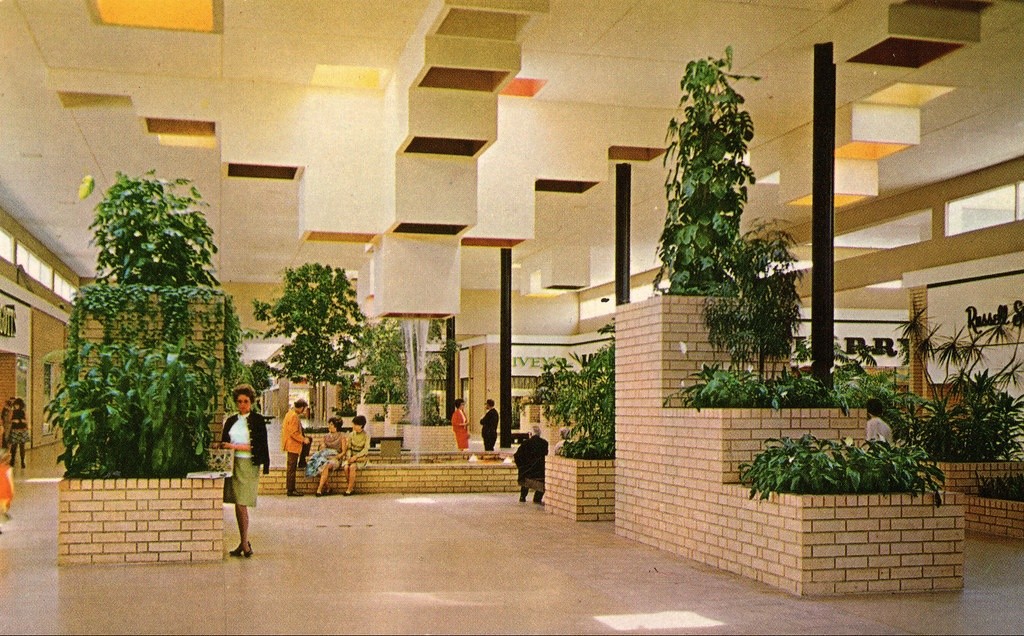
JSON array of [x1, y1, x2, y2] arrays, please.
[[244, 541, 253, 557], [324, 488, 333, 495], [229, 544, 243, 555], [520, 497, 525, 502], [286, 488, 304, 496], [316, 489, 321, 497], [344, 488, 355, 495]]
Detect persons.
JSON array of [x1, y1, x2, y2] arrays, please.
[[212, 384, 270, 557], [307, 416, 348, 497], [554, 427, 572, 457], [866, 398, 893, 444], [281, 399, 312, 497], [342, 415, 370, 496], [0, 397, 31, 535], [451, 398, 471, 452], [480, 399, 499, 451], [514, 426, 548, 505]]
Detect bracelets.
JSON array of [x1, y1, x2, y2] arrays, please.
[[18, 418, 22, 424]]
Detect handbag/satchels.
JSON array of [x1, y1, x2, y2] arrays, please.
[[203, 441, 235, 472]]
